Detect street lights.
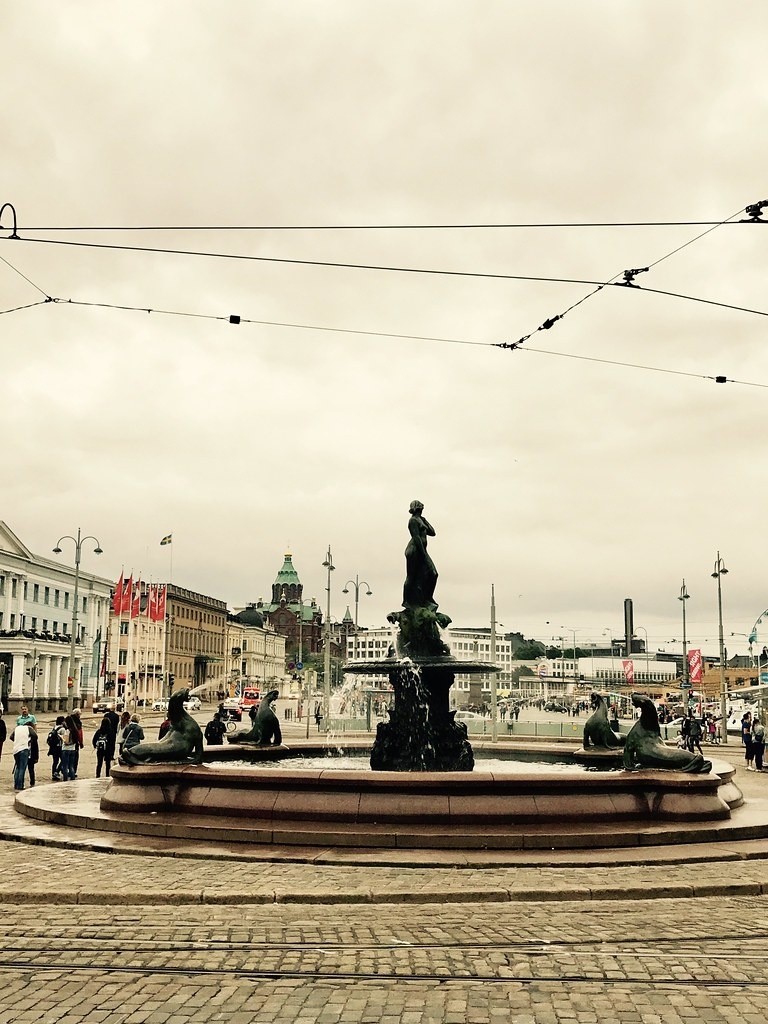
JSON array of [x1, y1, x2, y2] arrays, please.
[[677, 585, 691, 718], [631, 627, 650, 698], [322, 550, 336, 733], [602, 628, 616, 716], [342, 581, 372, 718], [53, 536, 104, 716], [711, 558, 729, 742]]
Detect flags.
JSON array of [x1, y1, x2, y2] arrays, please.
[[160, 535, 172, 545], [114, 570, 167, 623]]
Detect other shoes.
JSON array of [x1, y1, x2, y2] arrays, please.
[[745, 766, 755, 772], [15, 787, 26, 792], [52, 771, 60, 780], [67, 771, 77, 777], [756, 768, 765, 772]]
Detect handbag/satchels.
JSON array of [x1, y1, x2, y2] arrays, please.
[[755, 735, 762, 741], [710, 724, 715, 732]]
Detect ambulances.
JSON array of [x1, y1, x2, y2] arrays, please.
[[240, 687, 262, 711]]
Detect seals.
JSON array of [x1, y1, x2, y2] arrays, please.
[[583, 693, 627, 750], [623, 693, 712, 774], [118, 687, 203, 767], [227, 690, 282, 747]]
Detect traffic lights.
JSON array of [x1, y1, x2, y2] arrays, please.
[[25, 668, 33, 677], [35, 668, 43, 677]]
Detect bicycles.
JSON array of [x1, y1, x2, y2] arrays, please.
[[221, 715, 237, 732]]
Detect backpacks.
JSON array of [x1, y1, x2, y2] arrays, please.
[[68, 728, 79, 743], [47, 726, 62, 748], [96, 732, 109, 755], [116, 724, 127, 744]]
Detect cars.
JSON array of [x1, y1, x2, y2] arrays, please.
[[153, 697, 171, 710], [668, 718, 722, 739], [92, 698, 125, 714], [182, 697, 202, 710], [217, 702, 242, 722], [543, 703, 568, 715]]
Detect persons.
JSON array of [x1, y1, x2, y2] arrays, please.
[[314, 697, 395, 725], [218, 704, 224, 722], [741, 711, 765, 772], [0, 705, 144, 790], [204, 713, 226, 745], [216, 691, 229, 700], [458, 699, 589, 722], [158, 712, 171, 740], [608, 703, 619, 721], [250, 705, 276, 727], [404, 500, 438, 608], [657, 705, 719, 755]]
